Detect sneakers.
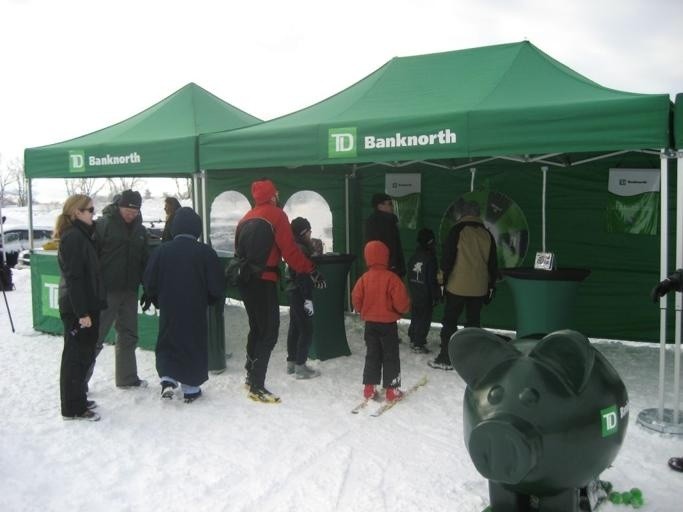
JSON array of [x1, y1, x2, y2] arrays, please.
[[161, 383, 174, 399], [386, 386, 403, 400], [407, 343, 453, 370], [364, 384, 376, 398], [295, 367, 321, 379], [287, 362, 295, 374], [184, 388, 201, 402], [117, 380, 147, 389], [86, 401, 95, 409], [248, 384, 280, 403], [64, 411, 99, 420]]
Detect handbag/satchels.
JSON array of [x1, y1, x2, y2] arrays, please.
[[225, 257, 251, 286]]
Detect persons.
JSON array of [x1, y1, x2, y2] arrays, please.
[[233, 180, 325, 402], [95, 190, 148, 389], [430, 200, 499, 369], [652, 268, 682, 473], [363, 192, 406, 343], [51, 195, 105, 421], [408, 227, 441, 351], [283, 217, 327, 378], [352, 240, 410, 402], [141, 207, 225, 401], [161, 197, 180, 237]]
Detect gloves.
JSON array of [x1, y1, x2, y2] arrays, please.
[[485, 287, 494, 305], [440, 284, 446, 303], [141, 294, 151, 310], [304, 299, 314, 319], [310, 269, 327, 289], [432, 297, 439, 307]]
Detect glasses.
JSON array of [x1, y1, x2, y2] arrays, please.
[[82, 207, 93, 212]]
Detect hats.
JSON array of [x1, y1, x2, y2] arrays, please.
[[417, 229, 435, 248], [372, 194, 390, 207], [252, 180, 275, 204], [291, 217, 311, 236], [463, 201, 480, 217], [120, 189, 141, 208]]
